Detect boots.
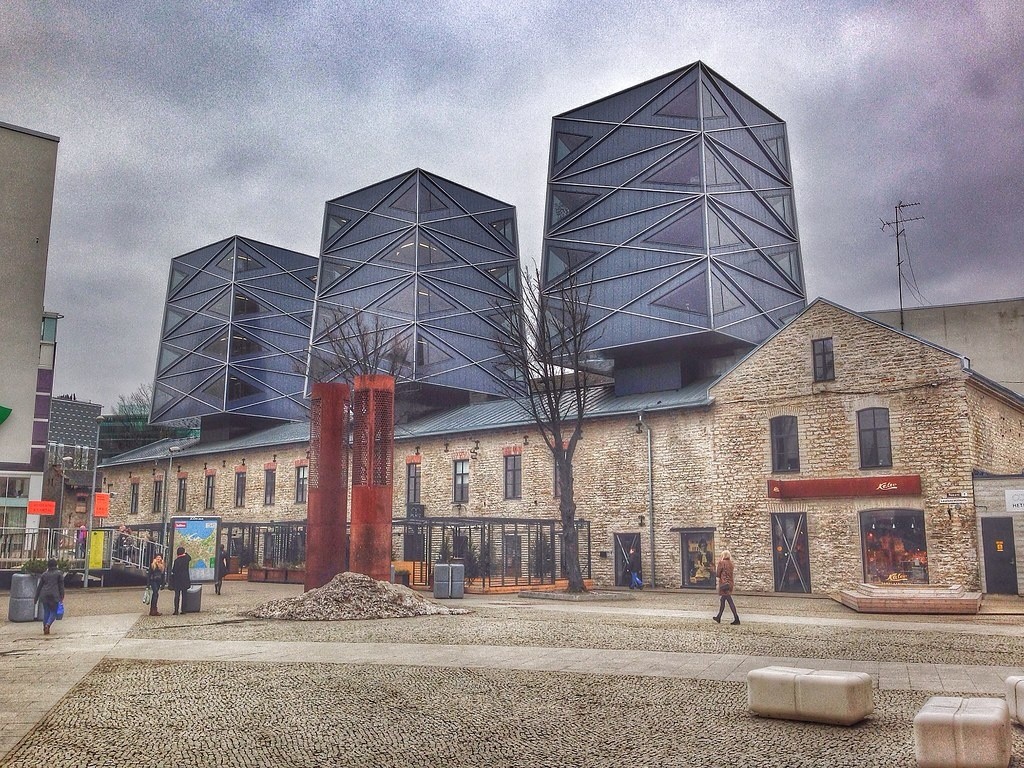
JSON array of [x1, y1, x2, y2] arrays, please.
[[150, 607, 162, 616]]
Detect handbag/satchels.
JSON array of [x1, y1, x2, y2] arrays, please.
[[721, 583, 729, 590], [142, 587, 151, 604], [167, 573, 174, 590], [56, 601, 63, 620]]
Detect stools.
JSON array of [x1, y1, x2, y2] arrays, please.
[[913, 695, 1012, 768], [1006, 676, 1024, 725]]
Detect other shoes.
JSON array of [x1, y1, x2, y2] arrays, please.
[[44, 624, 49, 635], [712, 616, 720, 622], [730, 619, 740, 625]]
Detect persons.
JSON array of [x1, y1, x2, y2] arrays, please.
[[79, 525, 87, 558], [115, 525, 157, 569], [171, 547, 191, 615], [627, 548, 644, 590], [696, 538, 714, 573], [218, 544, 229, 595], [33, 558, 65, 634], [713, 549, 741, 625], [146, 554, 165, 616]]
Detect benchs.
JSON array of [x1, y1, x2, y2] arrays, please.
[[746, 665, 875, 726]]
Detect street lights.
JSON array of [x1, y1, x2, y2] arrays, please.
[[159, 446, 180, 588], [80, 415, 106, 590], [55, 456, 73, 568]]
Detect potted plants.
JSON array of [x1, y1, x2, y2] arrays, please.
[[395, 569, 412, 586]]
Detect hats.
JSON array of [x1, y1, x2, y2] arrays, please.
[[48, 560, 57, 567]]
